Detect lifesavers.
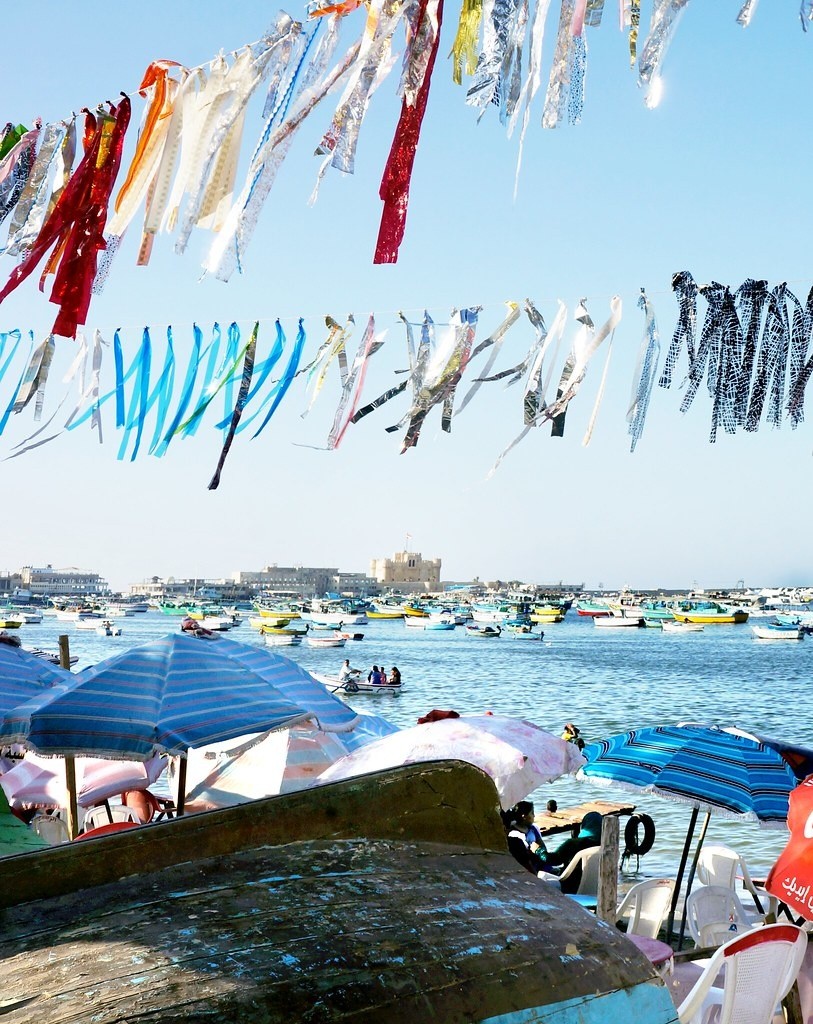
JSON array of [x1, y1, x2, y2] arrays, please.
[[624, 813, 656, 855]]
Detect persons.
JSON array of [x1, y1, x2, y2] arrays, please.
[[547, 800, 557, 812], [368, 665, 382, 684], [379, 667, 386, 684], [505, 802, 552, 873], [562, 723, 585, 751], [388, 667, 401, 684], [530, 811, 603, 894], [100, 621, 111, 628], [339, 659, 361, 681]]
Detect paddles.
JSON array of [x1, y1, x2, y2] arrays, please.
[[331, 668, 367, 694], [328, 670, 362, 676]]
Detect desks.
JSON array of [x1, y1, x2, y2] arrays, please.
[[736, 876, 806, 927]]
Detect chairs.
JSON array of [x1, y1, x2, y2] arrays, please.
[[535, 844, 807, 1024], [33, 789, 177, 845]]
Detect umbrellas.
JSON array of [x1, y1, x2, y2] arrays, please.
[[579, 722, 813, 950], [0, 632, 400, 841], [311, 714, 587, 813]]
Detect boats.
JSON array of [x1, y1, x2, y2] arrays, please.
[[333, 628, 364, 641], [0, 758, 682, 1023], [308, 671, 405, 697], [265, 630, 304, 646], [1, 586, 813, 642], [306, 635, 347, 648]]
